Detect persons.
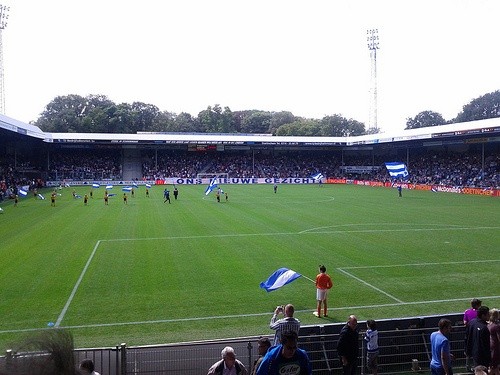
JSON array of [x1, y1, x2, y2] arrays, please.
[[49, 150, 122, 181], [464, 298, 500, 375], [269, 303, 301, 346], [313, 265, 332, 318], [123, 193, 127, 204], [253, 338, 271, 375], [0, 154, 44, 199], [53, 180, 78, 200], [217, 193, 220, 202], [146, 188, 149, 197], [142, 151, 342, 178], [362, 320, 379, 375], [51, 193, 56, 206], [164, 189, 171, 204], [430, 318, 455, 375], [256, 330, 313, 375], [131, 189, 134, 196], [397, 185, 402, 197], [319, 180, 322, 186], [345, 154, 500, 189], [84, 194, 88, 205], [337, 314, 359, 375], [216, 186, 225, 196], [104, 191, 108, 204], [14, 195, 18, 206], [207, 346, 249, 375], [274, 183, 277, 194], [225, 192, 228, 200], [79, 359, 100, 375], [173, 188, 178, 199]]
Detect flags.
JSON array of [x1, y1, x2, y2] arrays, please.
[[385, 161, 410, 178], [205, 176, 217, 196], [75, 195, 82, 198], [146, 184, 151, 188], [21, 185, 29, 192], [431, 188, 436, 193], [122, 186, 132, 192], [38, 194, 45, 200], [130, 183, 138, 188], [106, 183, 113, 190], [260, 267, 302, 293], [18, 188, 27, 198], [108, 193, 117, 197], [310, 172, 324, 181], [92, 182, 100, 188]]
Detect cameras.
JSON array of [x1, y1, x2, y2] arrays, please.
[[276, 305, 284, 312]]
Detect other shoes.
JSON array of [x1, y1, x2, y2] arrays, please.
[[323, 313, 328, 317], [314, 314, 320, 317]]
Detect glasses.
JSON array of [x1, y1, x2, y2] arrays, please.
[[286, 345, 297, 350]]
